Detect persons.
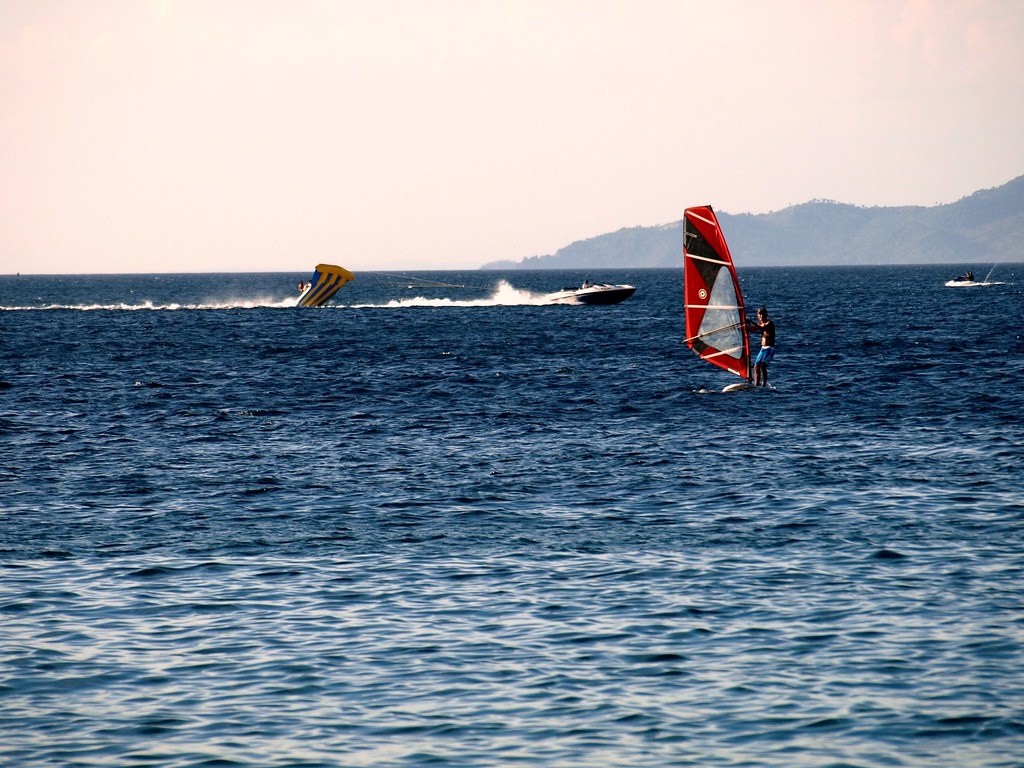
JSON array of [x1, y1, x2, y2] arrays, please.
[[582, 279, 589, 289], [298, 280, 312, 294], [730, 307, 776, 387]]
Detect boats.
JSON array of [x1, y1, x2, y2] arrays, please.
[[944, 279, 1007, 287], [535, 283, 638, 305]]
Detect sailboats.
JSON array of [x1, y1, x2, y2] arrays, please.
[[296, 263, 358, 308], [680, 204, 778, 396]]
